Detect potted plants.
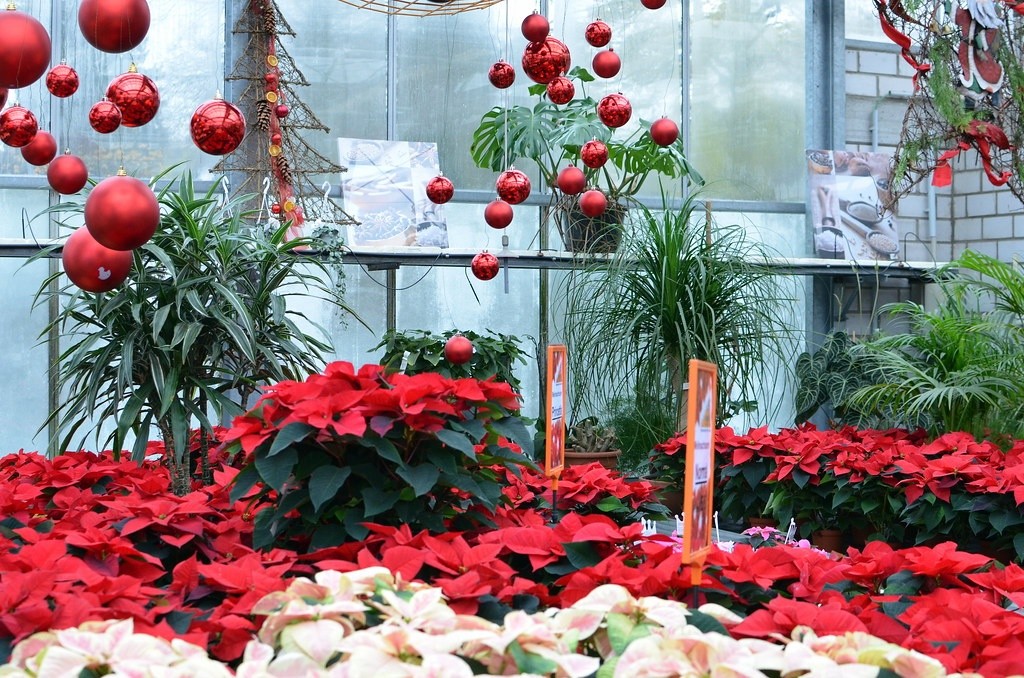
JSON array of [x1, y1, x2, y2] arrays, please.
[[563, 418, 622, 472], [472, 65, 707, 252], [560, 185, 834, 513]]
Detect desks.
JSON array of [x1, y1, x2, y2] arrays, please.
[[0, 236, 958, 461]]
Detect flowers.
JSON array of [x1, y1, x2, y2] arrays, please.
[[0, 357, 1024, 678]]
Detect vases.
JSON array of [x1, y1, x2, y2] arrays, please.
[[796, 519, 811, 540], [923, 535, 959, 546], [979, 539, 1013, 565], [851, 527, 874, 545], [748, 517, 776, 528], [811, 530, 846, 552]]
